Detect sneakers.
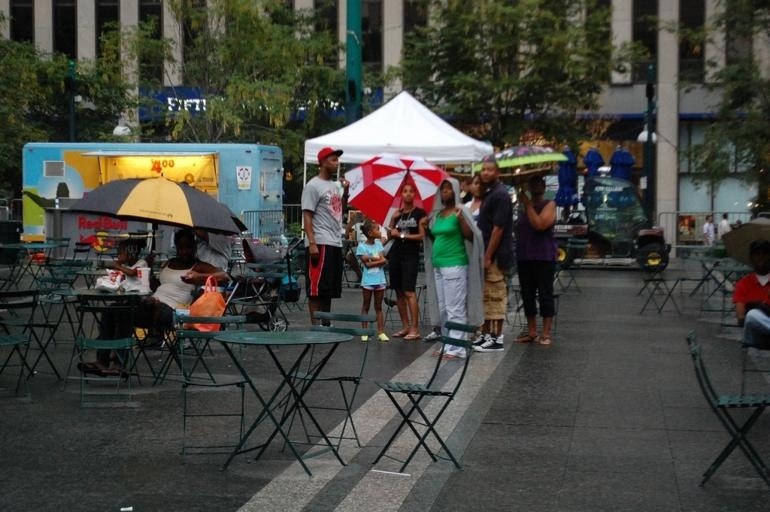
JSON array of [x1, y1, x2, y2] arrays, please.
[[375, 333, 390, 342], [421, 332, 443, 342], [437, 353, 463, 361], [432, 351, 442, 357], [474, 334, 505, 353], [360, 335, 369, 342], [471, 334, 487, 349]]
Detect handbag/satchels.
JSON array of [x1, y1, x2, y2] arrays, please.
[[381, 236, 396, 260], [181, 273, 230, 332]]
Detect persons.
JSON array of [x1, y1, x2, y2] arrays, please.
[[302, 148, 351, 328], [732, 238, 770, 350], [190, 225, 236, 274], [678, 212, 743, 248], [99, 236, 152, 295], [343, 177, 484, 361], [513, 174, 558, 347], [77, 231, 232, 382], [470, 159, 512, 353]]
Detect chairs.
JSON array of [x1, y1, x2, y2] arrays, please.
[[635, 256, 770, 487]]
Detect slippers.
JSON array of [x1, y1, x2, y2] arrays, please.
[[101, 367, 129, 377], [391, 331, 408, 338], [403, 335, 421, 340], [77, 361, 107, 378]]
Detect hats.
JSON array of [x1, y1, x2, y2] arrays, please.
[[316, 146, 344, 164]]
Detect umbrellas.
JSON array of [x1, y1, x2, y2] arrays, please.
[[720, 216, 770, 267], [555, 146, 579, 221], [476, 144, 569, 197], [70, 176, 245, 250], [582, 145, 605, 223], [607, 144, 637, 214], [343, 154, 454, 225]]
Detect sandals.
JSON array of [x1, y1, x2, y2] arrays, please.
[[512, 334, 539, 344], [539, 335, 552, 345]]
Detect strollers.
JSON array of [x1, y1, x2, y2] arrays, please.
[[228, 234, 304, 334]]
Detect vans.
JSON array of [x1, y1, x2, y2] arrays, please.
[[504, 170, 670, 273]]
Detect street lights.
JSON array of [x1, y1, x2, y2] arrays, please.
[[641, 20, 659, 224], [64, 60, 79, 143]]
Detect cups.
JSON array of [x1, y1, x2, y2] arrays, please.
[[137, 267, 150, 288], [55, 199, 59, 208]]
